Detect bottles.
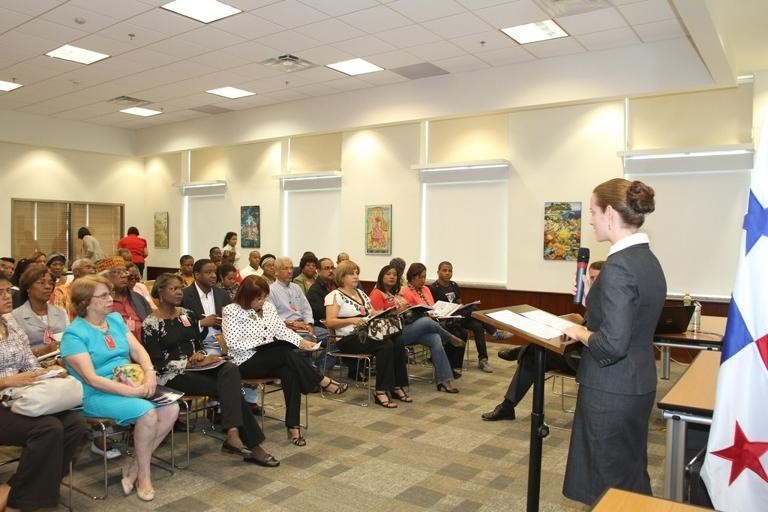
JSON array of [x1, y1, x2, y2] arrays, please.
[[692, 301, 701, 331], [684, 293, 692, 306]]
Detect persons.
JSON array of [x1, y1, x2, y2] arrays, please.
[[224, 274, 351, 447], [0, 270, 87, 512], [480, 260, 609, 422], [60, 274, 180, 502], [139, 271, 279, 467], [563, 177, 668, 506], [1, 247, 492, 411], [221, 231, 241, 270], [78, 227, 108, 264], [117, 226, 149, 274]]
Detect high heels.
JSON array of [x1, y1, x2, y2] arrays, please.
[[449, 335, 465, 347], [319, 378, 348, 394], [390, 386, 412, 403], [287, 427, 306, 446], [373, 390, 397, 408], [437, 382, 459, 393]]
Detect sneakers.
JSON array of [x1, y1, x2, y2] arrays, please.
[[493, 329, 514, 340], [478, 357, 493, 373]]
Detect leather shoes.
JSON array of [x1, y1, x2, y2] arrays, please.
[[121, 463, 156, 501], [498, 346, 522, 361], [482, 404, 515, 420], [206, 401, 280, 468], [349, 367, 376, 381], [174, 421, 195, 432], [90, 439, 122, 460]]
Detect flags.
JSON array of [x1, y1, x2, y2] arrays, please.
[[697, 121, 767, 512]]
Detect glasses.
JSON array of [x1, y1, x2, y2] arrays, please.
[[90, 290, 116, 299]]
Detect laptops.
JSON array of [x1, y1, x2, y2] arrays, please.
[[654, 305, 696, 334]]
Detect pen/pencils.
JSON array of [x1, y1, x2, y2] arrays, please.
[[201, 314, 223, 320], [431, 311, 439, 322], [49, 337, 56, 341]]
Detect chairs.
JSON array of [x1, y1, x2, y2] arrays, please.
[[318, 314, 375, 409], [55, 352, 176, 500], [214, 331, 311, 440]]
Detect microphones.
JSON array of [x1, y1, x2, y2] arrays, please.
[[575, 247, 590, 305]]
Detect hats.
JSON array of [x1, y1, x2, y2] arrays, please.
[[260, 254, 276, 268], [45, 251, 66, 266], [94, 256, 125, 272]]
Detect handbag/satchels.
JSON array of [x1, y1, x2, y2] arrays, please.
[[367, 315, 403, 341], [404, 309, 425, 324], [112, 363, 145, 386], [4, 375, 85, 417]]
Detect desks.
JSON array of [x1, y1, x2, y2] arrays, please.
[[654, 291, 728, 508]]
